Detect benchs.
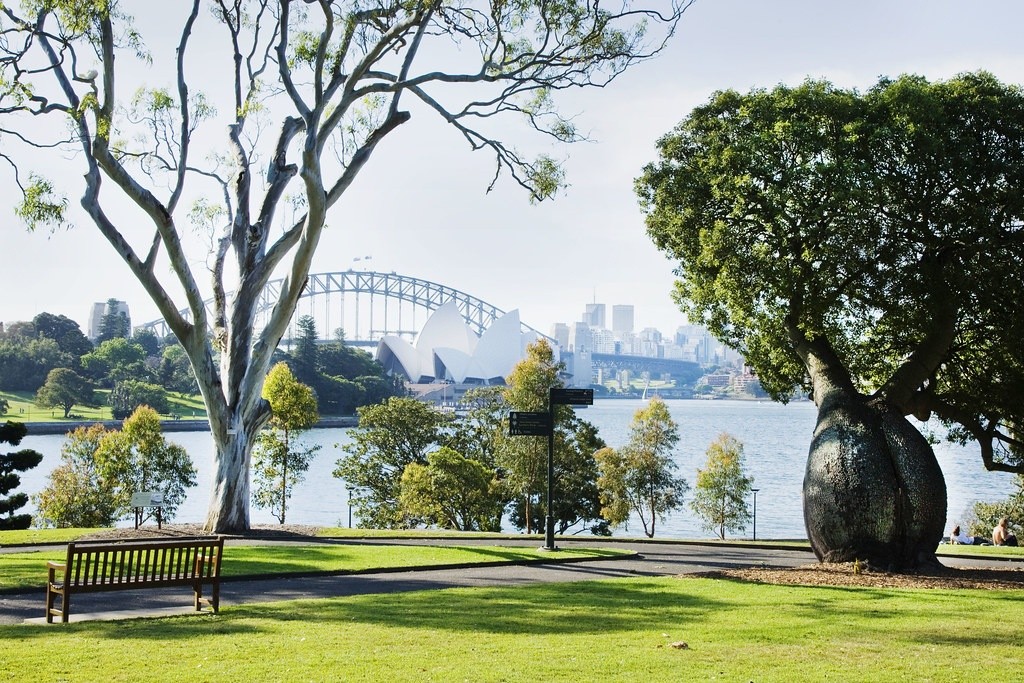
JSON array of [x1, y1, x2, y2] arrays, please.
[[47, 534, 227, 626]]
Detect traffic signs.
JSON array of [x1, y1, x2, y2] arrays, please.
[[510, 413, 549, 436], [548, 388, 594, 405]]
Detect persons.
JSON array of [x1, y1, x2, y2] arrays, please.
[[992, 518, 1018, 547], [950, 525, 993, 545]]
